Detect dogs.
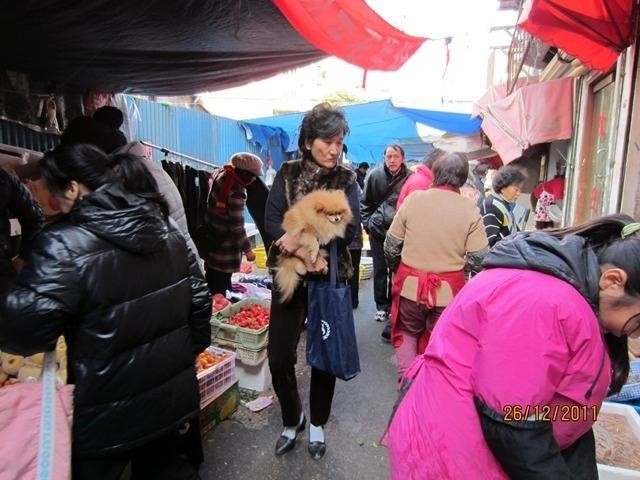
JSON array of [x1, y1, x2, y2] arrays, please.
[[273, 187, 354, 304]]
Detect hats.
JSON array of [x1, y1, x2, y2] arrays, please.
[[232, 152, 264, 177], [68, 105, 127, 155]]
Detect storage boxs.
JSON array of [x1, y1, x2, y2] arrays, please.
[[200, 381, 241, 436], [234, 356, 271, 391]]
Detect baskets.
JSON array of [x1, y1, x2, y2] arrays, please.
[[196, 346, 238, 403]]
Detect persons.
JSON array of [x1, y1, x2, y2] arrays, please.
[[355, 143, 526, 386], [1, 105, 211, 478], [382, 214, 640, 479], [265, 103, 360, 463], [205, 152, 263, 303], [531, 177, 564, 229]]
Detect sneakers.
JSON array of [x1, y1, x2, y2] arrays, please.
[[375, 310, 387, 322], [206, 296, 269, 366]]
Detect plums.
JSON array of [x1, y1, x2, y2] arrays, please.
[[210, 293, 229, 313]]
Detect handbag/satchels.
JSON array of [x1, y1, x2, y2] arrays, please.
[[194, 222, 228, 253], [305, 279, 360, 381]]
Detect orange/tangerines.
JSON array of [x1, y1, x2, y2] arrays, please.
[[197, 351, 226, 371]]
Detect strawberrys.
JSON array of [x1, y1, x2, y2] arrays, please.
[[228, 302, 270, 329]]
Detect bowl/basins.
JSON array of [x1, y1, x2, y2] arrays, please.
[[360, 256, 374, 280], [251, 248, 269, 269]]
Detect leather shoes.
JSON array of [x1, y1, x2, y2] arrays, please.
[[275, 414, 306, 455], [308, 423, 326, 461]]
